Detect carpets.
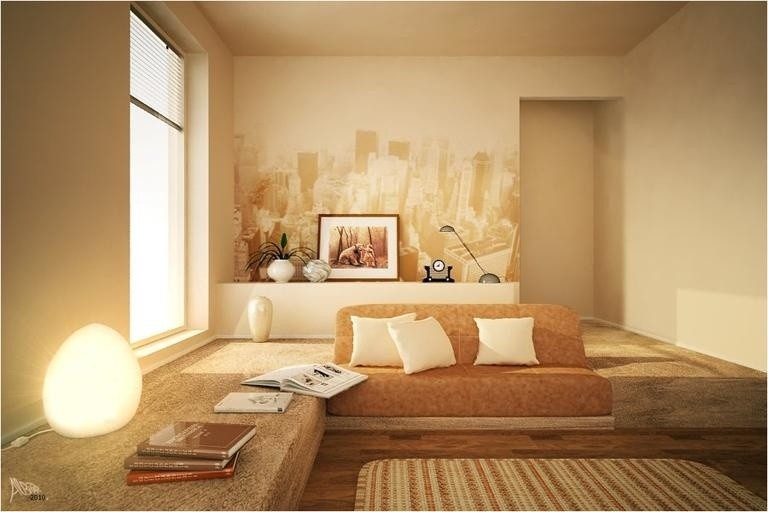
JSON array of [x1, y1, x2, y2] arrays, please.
[[353, 456, 765, 511]]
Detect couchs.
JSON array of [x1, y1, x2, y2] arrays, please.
[[325, 302, 617, 430]]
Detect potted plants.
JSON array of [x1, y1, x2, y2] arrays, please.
[[241, 232, 317, 282]]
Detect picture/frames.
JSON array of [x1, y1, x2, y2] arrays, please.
[[317, 213, 402, 281]]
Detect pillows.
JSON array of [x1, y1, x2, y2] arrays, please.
[[349, 311, 539, 376]]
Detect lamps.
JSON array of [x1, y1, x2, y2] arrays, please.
[[438, 225, 500, 283]]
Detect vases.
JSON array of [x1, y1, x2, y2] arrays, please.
[[248, 296, 273, 342]]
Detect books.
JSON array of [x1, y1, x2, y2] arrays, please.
[[239, 362, 369, 398], [136, 421, 257, 456], [123, 452, 233, 470], [214, 392, 293, 413], [126, 452, 240, 487]]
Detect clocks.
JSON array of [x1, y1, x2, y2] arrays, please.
[[422, 258, 455, 283]]
